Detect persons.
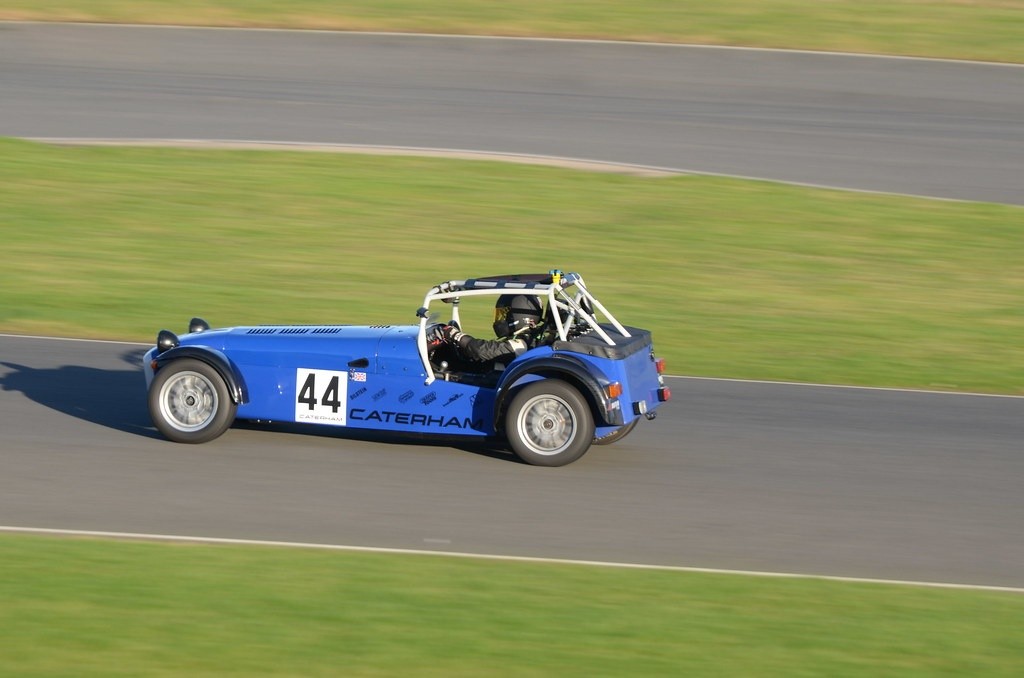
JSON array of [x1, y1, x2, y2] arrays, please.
[[443, 295, 544, 373]]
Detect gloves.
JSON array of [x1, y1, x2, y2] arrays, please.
[[443, 325, 462, 342]]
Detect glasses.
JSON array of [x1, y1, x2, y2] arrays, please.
[[495, 307, 508, 322]]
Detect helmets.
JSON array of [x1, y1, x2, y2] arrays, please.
[[493, 294, 542, 336]]
